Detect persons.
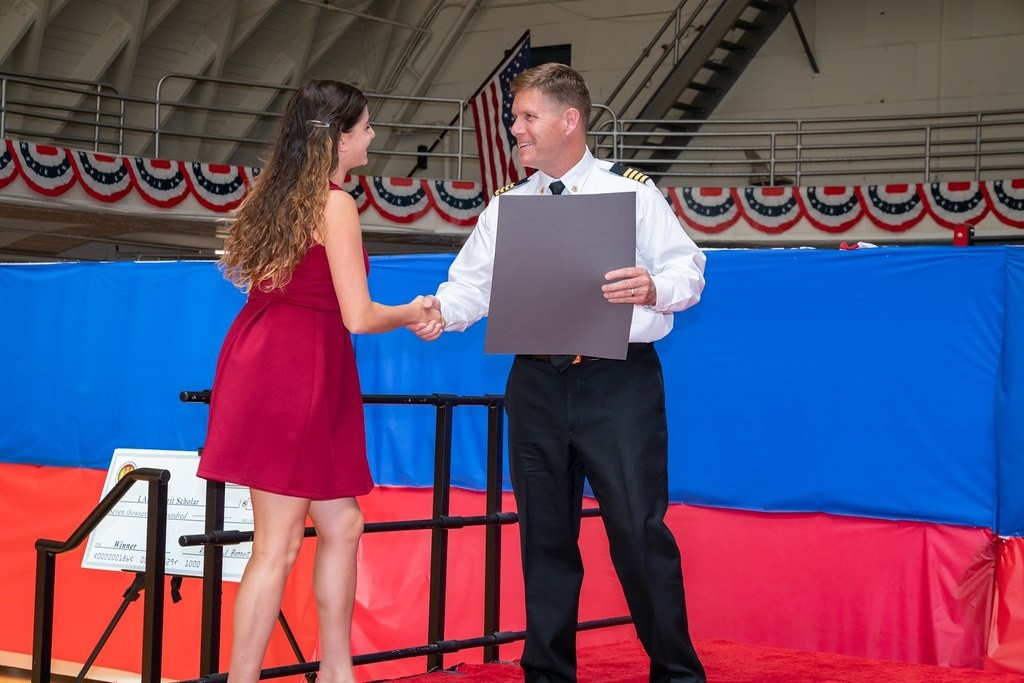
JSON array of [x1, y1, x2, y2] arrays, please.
[[405, 63, 707, 682], [197, 78, 443, 682]]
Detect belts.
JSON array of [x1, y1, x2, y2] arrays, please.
[[519, 342, 654, 364]]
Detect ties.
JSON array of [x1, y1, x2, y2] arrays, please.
[[549, 181, 572, 371]]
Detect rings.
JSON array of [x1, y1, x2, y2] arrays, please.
[[632, 289, 634, 296]]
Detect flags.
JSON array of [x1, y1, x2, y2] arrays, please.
[[472, 33, 538, 208]]
